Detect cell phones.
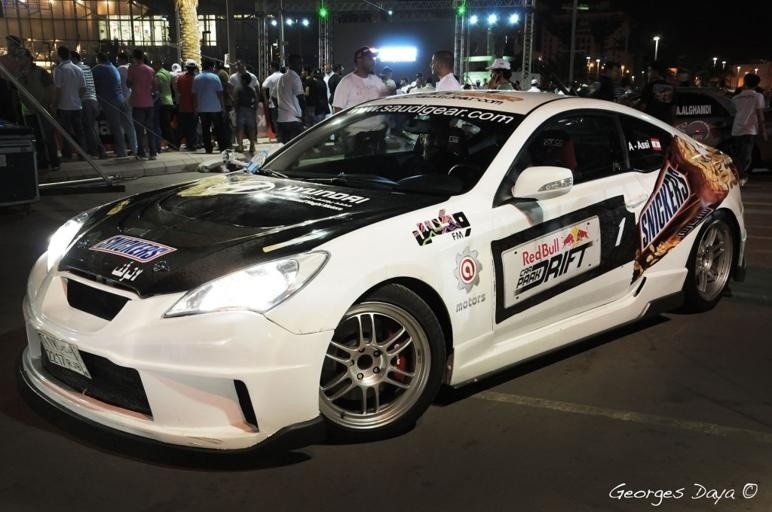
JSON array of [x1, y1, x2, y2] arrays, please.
[[495, 71, 503, 83]]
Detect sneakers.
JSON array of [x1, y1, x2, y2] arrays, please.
[[740, 177, 750, 187], [37, 141, 257, 173]]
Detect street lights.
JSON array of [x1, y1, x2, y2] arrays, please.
[[722, 60, 726, 70], [586, 56, 591, 72], [621, 65, 626, 77], [737, 66, 741, 75], [568, 4, 590, 83], [652, 35, 661, 61], [754, 69, 758, 76], [594, 58, 600, 78], [712, 57, 718, 68]]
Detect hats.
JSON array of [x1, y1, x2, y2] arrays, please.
[[484, 57, 512, 72], [530, 79, 542, 86], [353, 47, 379, 61], [185, 60, 197, 68]]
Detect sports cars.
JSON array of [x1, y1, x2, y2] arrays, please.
[[20, 86, 750, 452], [565, 84, 772, 174]]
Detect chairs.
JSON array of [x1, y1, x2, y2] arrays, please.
[[531, 130, 583, 181], [442, 127, 470, 163]]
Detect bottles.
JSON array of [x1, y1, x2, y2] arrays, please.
[[246, 149, 268, 173]]
